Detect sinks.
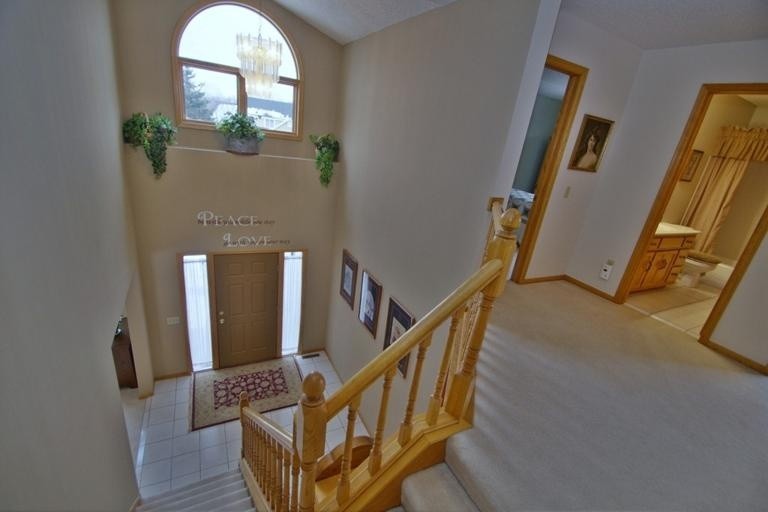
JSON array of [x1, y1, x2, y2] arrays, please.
[[657, 223, 680, 234]]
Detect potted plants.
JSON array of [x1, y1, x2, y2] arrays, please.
[[215, 110, 266, 156], [308, 132, 339, 189]]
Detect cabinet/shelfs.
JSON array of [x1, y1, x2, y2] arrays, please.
[[628, 235, 696, 292]]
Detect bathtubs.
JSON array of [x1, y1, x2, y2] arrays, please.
[[701, 254, 738, 289]]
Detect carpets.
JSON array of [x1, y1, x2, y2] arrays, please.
[[187, 353, 306, 431]]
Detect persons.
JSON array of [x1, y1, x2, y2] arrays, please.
[[573, 122, 603, 173]]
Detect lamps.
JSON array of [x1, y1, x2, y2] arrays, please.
[[235, 1, 283, 98]]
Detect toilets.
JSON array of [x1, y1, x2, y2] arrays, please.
[[680, 249, 722, 288]]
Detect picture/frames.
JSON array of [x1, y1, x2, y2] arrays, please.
[[566, 113, 616, 174], [340, 248, 415, 380], [678, 149, 705, 183]]
